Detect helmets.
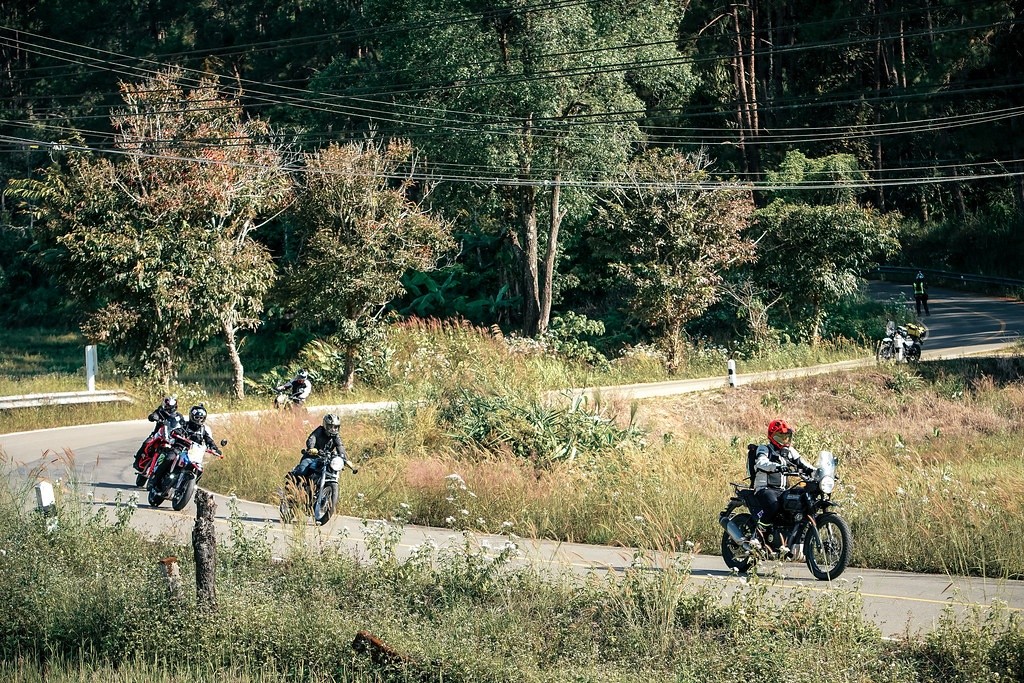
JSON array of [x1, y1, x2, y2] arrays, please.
[[162, 397, 178, 416], [768, 419, 793, 448], [297, 369, 308, 380], [189, 406, 207, 425], [916, 273, 924, 279], [323, 413, 341, 436]]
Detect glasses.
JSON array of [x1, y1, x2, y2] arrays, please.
[[194, 415, 204, 421], [773, 433, 791, 445], [326, 424, 338, 432]]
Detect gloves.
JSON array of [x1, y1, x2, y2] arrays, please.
[[174, 429, 181, 436], [216, 449, 222, 455], [152, 415, 159, 422], [776, 465, 792, 476], [308, 448, 318, 456]]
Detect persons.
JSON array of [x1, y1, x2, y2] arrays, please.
[[297, 414, 345, 475], [912, 272, 930, 317], [749, 419, 816, 550], [133, 397, 184, 467], [277, 370, 311, 405], [147, 406, 222, 488]]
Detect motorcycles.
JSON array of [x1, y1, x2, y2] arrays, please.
[[718, 450, 853, 581], [133, 415, 189, 491], [876, 319, 924, 367], [271, 378, 308, 417], [280, 448, 358, 526], [147, 429, 227, 511]]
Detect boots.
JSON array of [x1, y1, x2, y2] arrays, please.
[[749, 519, 773, 549]]
